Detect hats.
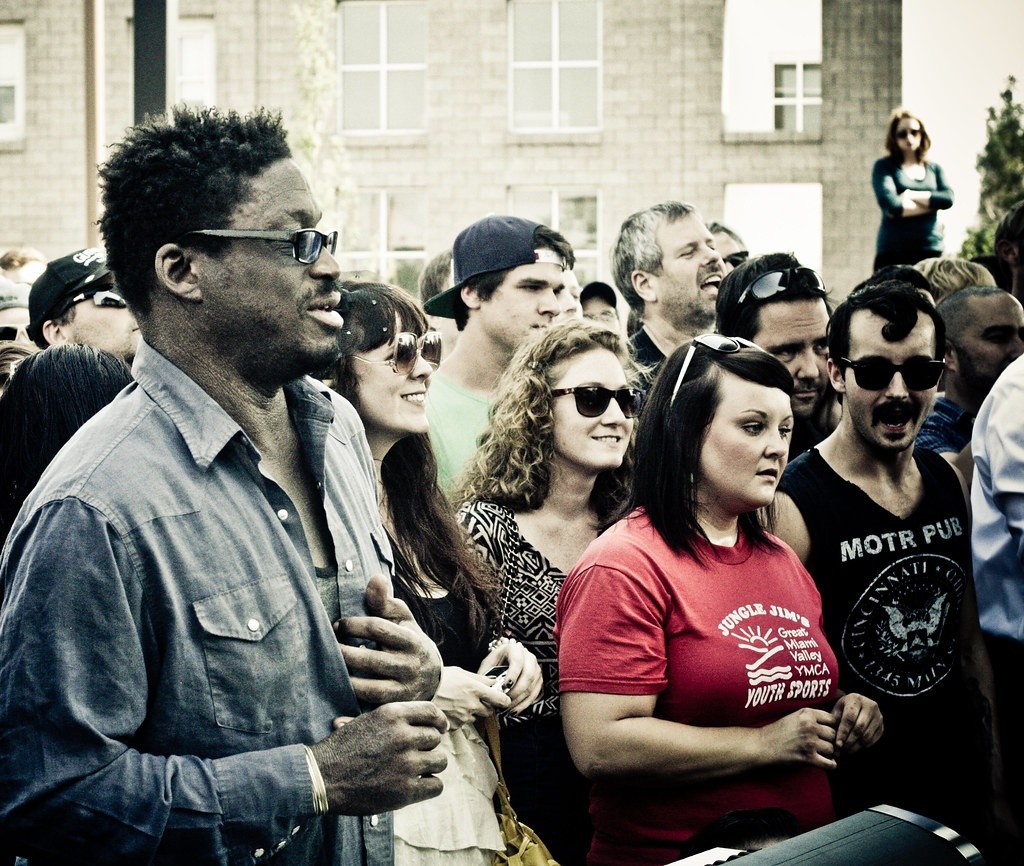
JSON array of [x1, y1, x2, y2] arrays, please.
[[24, 247, 110, 349], [578, 281, 616, 308], [0, 283, 33, 311], [423, 215, 568, 319]]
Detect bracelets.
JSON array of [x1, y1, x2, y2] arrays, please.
[[304, 745, 329, 818]]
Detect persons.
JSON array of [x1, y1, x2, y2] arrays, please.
[[446, 317, 658, 866], [609, 200, 842, 464], [912, 200, 1024, 502], [871, 110, 954, 272], [309, 281, 543, 865], [0, 248, 46, 398], [971, 353, 1024, 866], [552, 333, 882, 866], [28, 246, 141, 373], [0, 344, 134, 555], [756, 279, 1024, 866], [420, 216, 621, 498], [0, 105, 450, 865]]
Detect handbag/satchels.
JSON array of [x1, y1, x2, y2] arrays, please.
[[472, 703, 562, 866]]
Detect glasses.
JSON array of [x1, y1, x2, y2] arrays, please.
[[736, 267, 826, 307], [721, 251, 749, 268], [896, 129, 922, 138], [345, 331, 441, 376], [175, 229, 338, 266], [54, 283, 127, 319], [0, 324, 36, 343], [670, 333, 767, 408], [840, 355, 945, 391], [551, 386, 647, 418]]
